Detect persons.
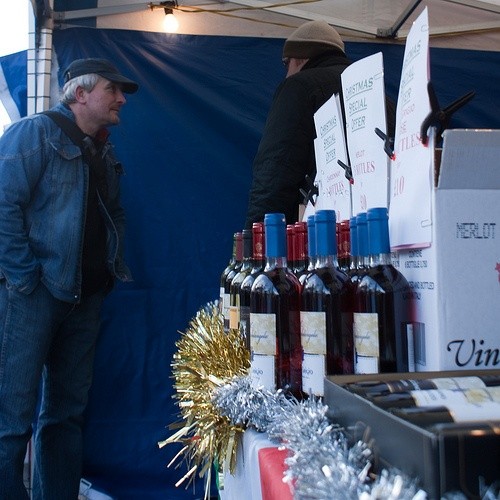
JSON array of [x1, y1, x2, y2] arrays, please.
[[238, 15, 397, 229], [0, 57, 139, 500]]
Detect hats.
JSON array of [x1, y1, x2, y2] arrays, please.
[[63, 59, 138, 95], [283, 20, 345, 60]]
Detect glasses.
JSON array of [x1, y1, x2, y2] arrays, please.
[[283, 57, 293, 67]]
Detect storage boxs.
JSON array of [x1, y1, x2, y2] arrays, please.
[[391, 128, 500, 373], [324, 367, 500, 500]]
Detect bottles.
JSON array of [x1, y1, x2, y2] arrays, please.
[[343, 372, 500, 436], [220, 207, 416, 405]]
